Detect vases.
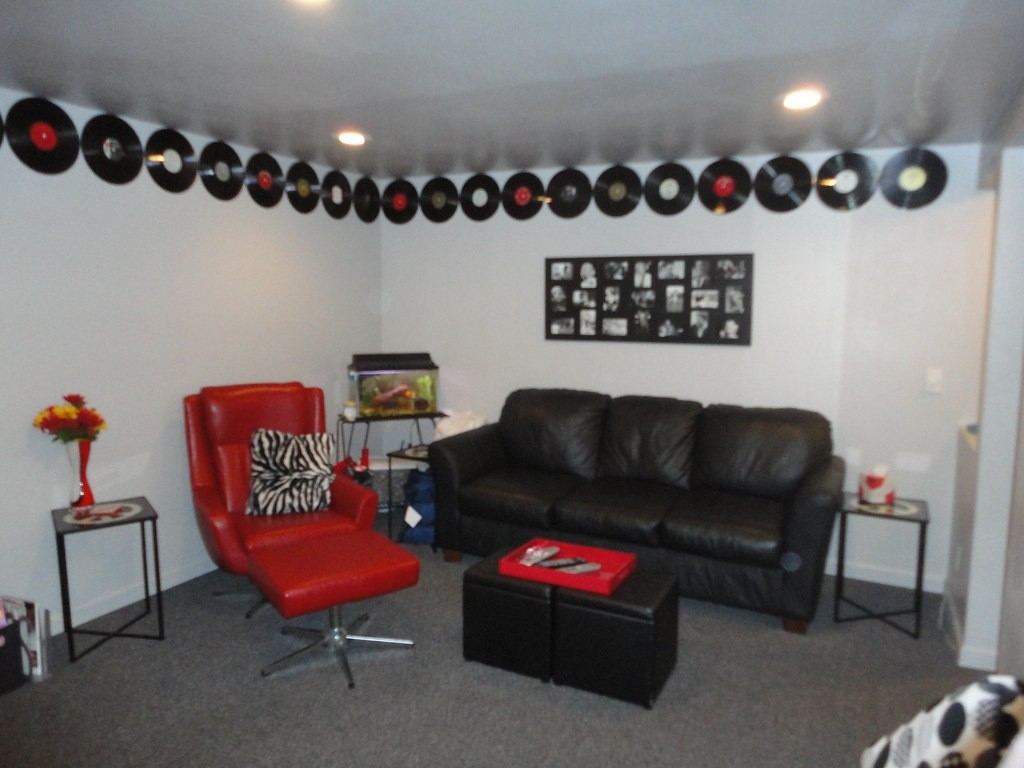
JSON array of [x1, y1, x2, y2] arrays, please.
[[64, 439, 97, 509]]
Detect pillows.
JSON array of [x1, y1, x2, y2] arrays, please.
[[245, 429, 336, 515]]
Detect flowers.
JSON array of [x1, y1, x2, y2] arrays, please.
[[32, 394, 100, 442]]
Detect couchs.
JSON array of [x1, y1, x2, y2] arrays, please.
[[427, 388, 847, 636]]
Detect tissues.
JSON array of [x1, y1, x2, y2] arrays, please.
[[859, 464, 896, 505]]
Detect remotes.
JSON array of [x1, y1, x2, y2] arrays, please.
[[557, 562, 601, 574], [520, 546, 559, 567], [539, 557, 587, 568]]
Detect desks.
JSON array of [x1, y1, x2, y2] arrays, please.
[[385, 444, 429, 541], [53, 496, 165, 661], [833, 492, 931, 640], [336, 407, 448, 540]]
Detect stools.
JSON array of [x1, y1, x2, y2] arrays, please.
[[557, 575, 680, 710], [245, 524, 421, 689], [463, 544, 556, 684]]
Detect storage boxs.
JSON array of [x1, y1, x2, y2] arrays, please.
[[348, 353, 437, 418]]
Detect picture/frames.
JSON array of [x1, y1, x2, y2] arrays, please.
[[544, 254, 753, 346]]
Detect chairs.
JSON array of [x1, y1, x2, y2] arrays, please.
[[183, 381, 379, 622]]
